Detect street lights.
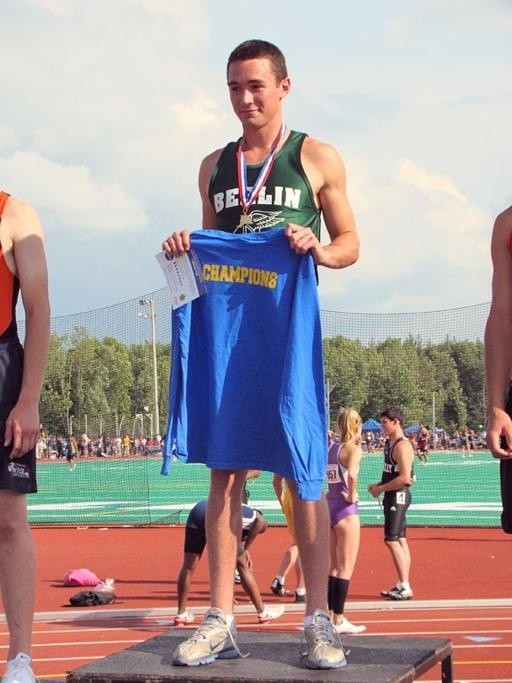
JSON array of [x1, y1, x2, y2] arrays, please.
[[137, 297, 162, 439]]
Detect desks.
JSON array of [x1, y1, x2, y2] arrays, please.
[[66, 628, 453, 683]]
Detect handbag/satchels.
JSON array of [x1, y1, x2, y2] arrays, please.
[[63, 568, 103, 588], [68, 590, 117, 605]]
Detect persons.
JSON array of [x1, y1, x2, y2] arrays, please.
[[230, 470, 260, 584], [484, 204, 512, 538], [159, 39, 359, 671], [173, 499, 272, 625], [367, 406, 414, 600], [270, 472, 307, 604], [0, 190, 50, 683], [325, 409, 366, 636]]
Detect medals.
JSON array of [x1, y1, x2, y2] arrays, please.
[[239, 207, 252, 224]]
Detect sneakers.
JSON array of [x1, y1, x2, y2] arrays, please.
[[301, 612, 347, 671], [381, 583, 415, 599], [294, 590, 307, 603], [271, 577, 286, 596], [257, 608, 284, 624], [0, 653, 36, 682], [234, 568, 242, 585], [174, 611, 196, 628], [172, 606, 243, 668], [331, 614, 366, 633]]
